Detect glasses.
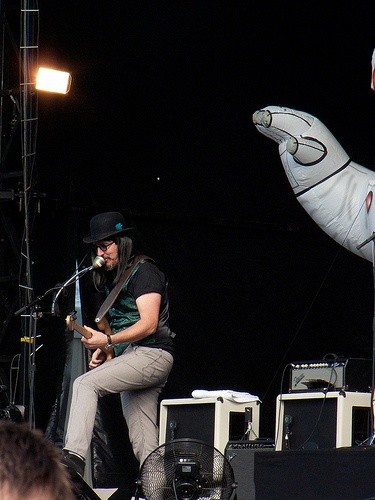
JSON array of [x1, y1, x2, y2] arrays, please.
[[92, 241, 115, 251]]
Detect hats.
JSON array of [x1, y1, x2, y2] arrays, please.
[[83, 212, 138, 243]]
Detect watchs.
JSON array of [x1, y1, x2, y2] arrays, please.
[[105, 335, 112, 351]]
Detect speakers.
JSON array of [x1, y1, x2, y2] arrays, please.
[[224, 445, 375, 500]]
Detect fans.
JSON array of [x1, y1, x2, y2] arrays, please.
[[135, 438, 239, 500]]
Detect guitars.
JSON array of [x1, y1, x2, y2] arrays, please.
[[64, 314, 115, 360]]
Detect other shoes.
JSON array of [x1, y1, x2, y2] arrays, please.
[[57, 454, 85, 477]]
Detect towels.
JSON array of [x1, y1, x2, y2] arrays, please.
[[191, 389, 263, 404]]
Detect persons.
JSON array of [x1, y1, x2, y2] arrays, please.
[[0, 421, 74, 500], [56, 212, 176, 500]]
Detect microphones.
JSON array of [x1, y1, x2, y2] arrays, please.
[[91, 255, 105, 269]]
